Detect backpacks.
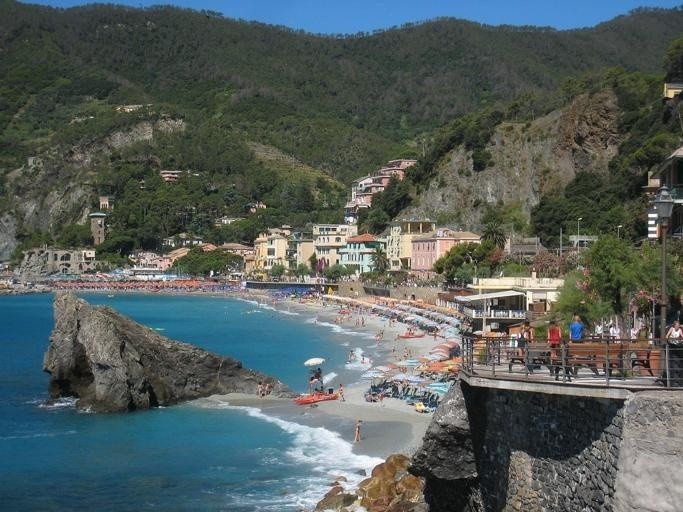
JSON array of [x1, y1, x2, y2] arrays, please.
[[518, 336, 525, 348]]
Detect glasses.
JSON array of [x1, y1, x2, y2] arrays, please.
[[642, 325, 646, 327]]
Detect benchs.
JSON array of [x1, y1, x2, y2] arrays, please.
[[507, 343, 654, 382]]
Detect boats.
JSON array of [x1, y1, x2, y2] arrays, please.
[[293, 391, 339, 407]]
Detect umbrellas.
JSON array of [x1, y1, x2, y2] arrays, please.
[[304, 357, 325, 372]]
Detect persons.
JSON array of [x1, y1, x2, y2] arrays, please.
[[634, 323, 650, 344], [547, 319, 561, 358], [335, 316, 438, 373], [569, 315, 586, 344], [354, 420, 362, 442], [258, 382, 271, 400], [666, 321, 683, 346], [339, 384, 345, 402], [310, 367, 323, 395], [517, 320, 533, 364]]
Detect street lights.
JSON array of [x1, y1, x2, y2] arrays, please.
[[616, 224, 622, 237], [576, 215, 582, 268], [652, 182, 678, 385]]
